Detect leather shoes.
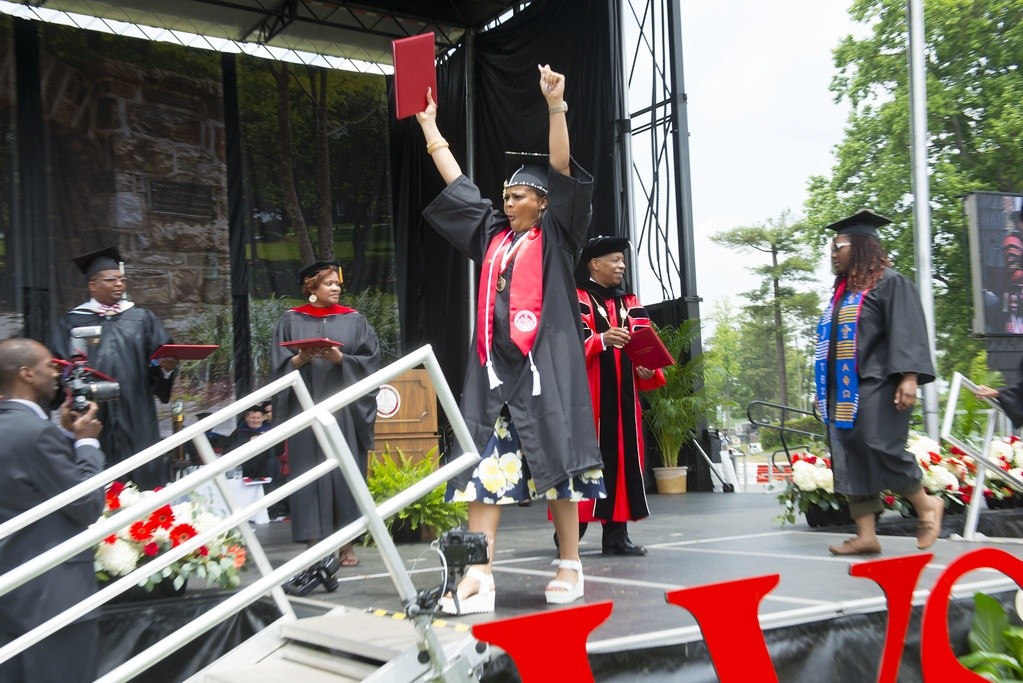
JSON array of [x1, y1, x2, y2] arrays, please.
[[601, 541, 646, 555]]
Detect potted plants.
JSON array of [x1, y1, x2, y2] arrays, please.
[[370, 447, 450, 545], [640, 323, 729, 493]]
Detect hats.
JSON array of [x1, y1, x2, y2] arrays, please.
[[72, 245, 126, 276], [826, 210, 893, 241], [298, 260, 344, 285], [579, 235, 628, 263], [501, 150, 550, 195], [247, 405, 268, 414]]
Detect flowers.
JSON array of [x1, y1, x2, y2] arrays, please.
[[97, 485, 242, 578], [794, 429, 1022, 489]]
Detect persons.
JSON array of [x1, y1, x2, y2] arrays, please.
[[998, 211, 1023, 333], [813, 210, 945, 556], [976, 358, 1023, 429], [50, 245, 179, 492], [416, 64, 595, 615], [548, 237, 668, 555], [269, 259, 380, 567], [0, 337, 106, 683], [186, 401, 291, 516]]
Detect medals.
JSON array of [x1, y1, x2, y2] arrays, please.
[[496, 276, 506, 291]]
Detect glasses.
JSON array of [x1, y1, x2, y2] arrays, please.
[[829, 242, 852, 250]]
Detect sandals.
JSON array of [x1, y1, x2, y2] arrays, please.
[[338, 549, 360, 566]]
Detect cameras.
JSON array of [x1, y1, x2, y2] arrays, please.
[[438, 527, 490, 567], [65, 378, 120, 415]]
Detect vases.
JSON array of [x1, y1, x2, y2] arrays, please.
[[807, 494, 1021, 525], [118, 572, 187, 599]]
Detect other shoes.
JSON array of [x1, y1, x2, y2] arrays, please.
[[917, 495, 944, 549], [829, 536, 881, 554]]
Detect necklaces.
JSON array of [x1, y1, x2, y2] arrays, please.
[[588, 293, 627, 348]]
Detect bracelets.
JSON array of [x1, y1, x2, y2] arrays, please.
[[427, 137, 449, 155]]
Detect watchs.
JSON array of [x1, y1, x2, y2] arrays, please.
[[548, 101, 568, 114]]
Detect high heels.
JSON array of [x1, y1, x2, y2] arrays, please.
[[545, 558, 584, 603], [439, 567, 496, 614]]
